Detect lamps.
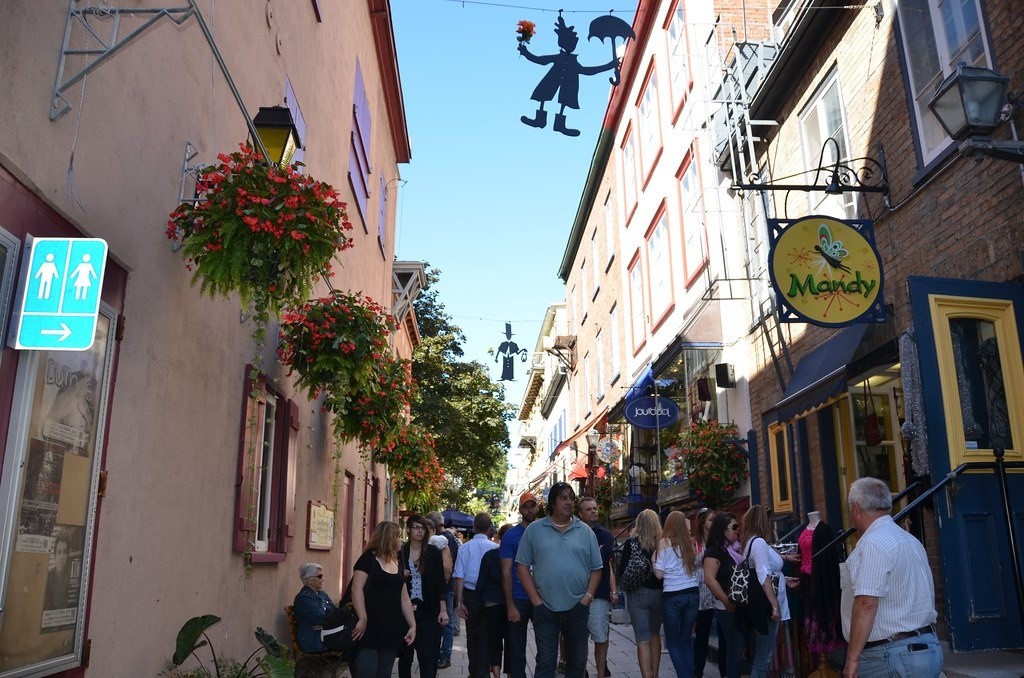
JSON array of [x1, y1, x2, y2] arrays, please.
[[927, 59, 1024, 164]]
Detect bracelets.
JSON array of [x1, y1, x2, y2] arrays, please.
[[773, 608, 780, 612], [585, 591, 595, 601], [612, 591, 617, 594], [457, 602, 463, 606], [784, 575, 788, 582], [786, 555, 788, 561]]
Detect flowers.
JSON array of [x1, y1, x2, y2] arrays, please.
[[163, 138, 449, 514], [515, 20, 537, 58], [665, 417, 752, 508], [594, 469, 627, 516]]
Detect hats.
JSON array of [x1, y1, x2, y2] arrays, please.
[[520, 492, 537, 506]]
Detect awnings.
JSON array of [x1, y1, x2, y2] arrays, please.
[[548, 404, 611, 478], [772, 322, 898, 426], [509, 454, 565, 512], [606, 273, 723, 425], [565, 454, 590, 480]]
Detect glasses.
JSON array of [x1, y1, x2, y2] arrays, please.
[[309, 574, 322, 579], [410, 525, 425, 530], [700, 507, 708, 515], [728, 524, 739, 530]]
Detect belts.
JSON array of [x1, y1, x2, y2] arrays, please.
[[862, 623, 937, 649]]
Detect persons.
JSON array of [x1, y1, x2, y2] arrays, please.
[[833, 478, 944, 678], [576, 496, 619, 678], [688, 505, 751, 678], [452, 509, 517, 678], [43, 337, 100, 445], [45, 533, 70, 610], [616, 507, 701, 678], [498, 482, 603, 678], [740, 499, 801, 678], [425, 510, 469, 670], [794, 508, 839, 644], [292, 512, 450, 678]]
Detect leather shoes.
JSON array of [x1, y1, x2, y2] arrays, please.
[[437, 659, 451, 668]]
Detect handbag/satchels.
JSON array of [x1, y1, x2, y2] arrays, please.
[[323, 607, 362, 650], [729, 536, 780, 608], [620, 537, 654, 592]]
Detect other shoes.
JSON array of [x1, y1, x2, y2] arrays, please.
[[558, 660, 566, 674]]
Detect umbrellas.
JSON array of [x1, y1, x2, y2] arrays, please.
[[458, 522, 493, 538], [442, 505, 474, 529]]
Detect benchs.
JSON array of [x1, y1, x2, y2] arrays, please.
[[284, 604, 346, 678]]
